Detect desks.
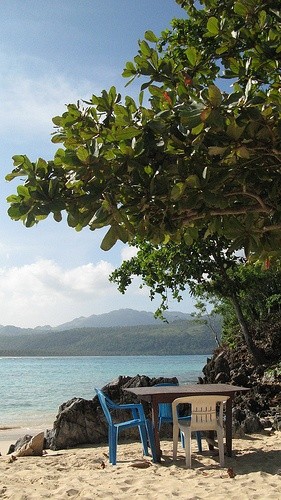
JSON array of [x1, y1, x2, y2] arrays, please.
[[122, 382, 251, 462]]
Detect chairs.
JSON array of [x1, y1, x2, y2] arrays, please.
[[154, 382, 203, 452], [94, 387, 157, 465], [171, 396, 231, 468]]
